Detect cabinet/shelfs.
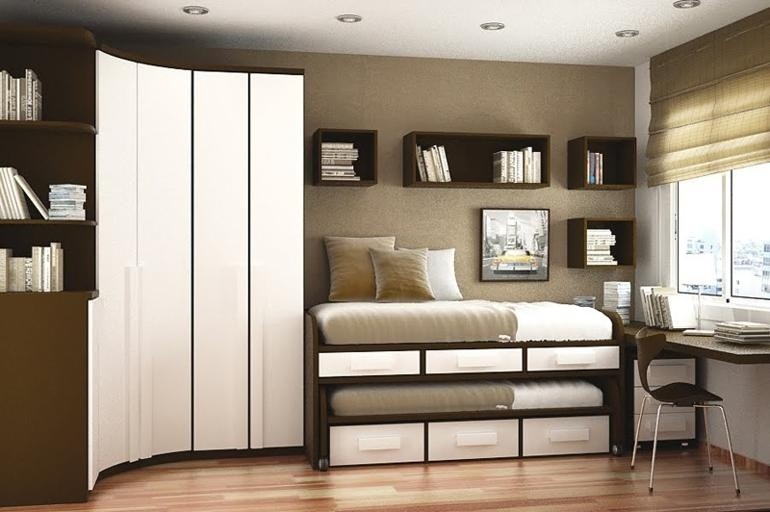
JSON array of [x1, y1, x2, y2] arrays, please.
[[0, 41, 304, 507], [304, 309, 631, 468]]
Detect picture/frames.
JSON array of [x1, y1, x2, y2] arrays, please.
[[481, 208, 550, 282]]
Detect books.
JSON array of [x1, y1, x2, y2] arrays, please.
[[14, 175, 49, 221], [0, 166, 31, 220], [416, 144, 452, 182], [48, 183, 87, 221], [0, 242, 64, 293], [602, 280, 632, 326], [587, 150, 604, 185], [0, 69, 43, 121], [492, 146, 542, 184], [640, 285, 697, 330], [713, 320, 770, 346], [587, 228, 618, 265], [321, 142, 360, 181]]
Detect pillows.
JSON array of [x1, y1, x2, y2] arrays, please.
[[324, 236, 463, 301]]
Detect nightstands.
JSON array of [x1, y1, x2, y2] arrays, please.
[[632, 358, 696, 447]]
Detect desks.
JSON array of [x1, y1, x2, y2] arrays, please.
[[624, 321, 770, 363], [629, 326, 741, 497]]
